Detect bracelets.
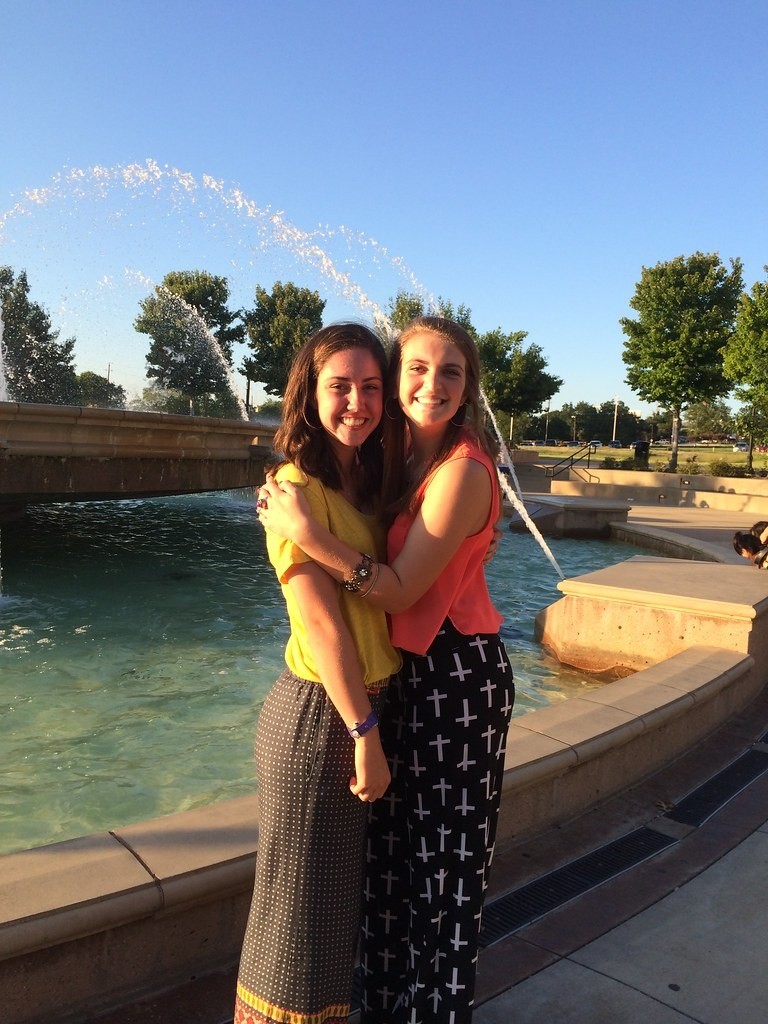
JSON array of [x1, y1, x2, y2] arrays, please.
[[341, 552, 381, 599], [346, 711, 379, 739]]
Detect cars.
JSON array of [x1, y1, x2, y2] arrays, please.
[[721, 438, 735, 443], [588, 440, 603, 449], [609, 440, 622, 450], [535, 439, 546, 447], [754, 444, 768, 453], [519, 440, 534, 447], [654, 439, 671, 445], [702, 439, 718, 444], [629, 442, 637, 450], [567, 440, 580, 448], [545, 439, 558, 446], [732, 442, 749, 452]]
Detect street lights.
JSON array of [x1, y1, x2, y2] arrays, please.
[[650, 423, 654, 440], [571, 416, 577, 441]]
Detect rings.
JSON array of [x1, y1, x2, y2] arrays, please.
[[256, 495, 269, 510]]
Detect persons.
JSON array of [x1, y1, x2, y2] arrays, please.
[[733, 521, 768, 570], [233, 316, 515, 1024]]
